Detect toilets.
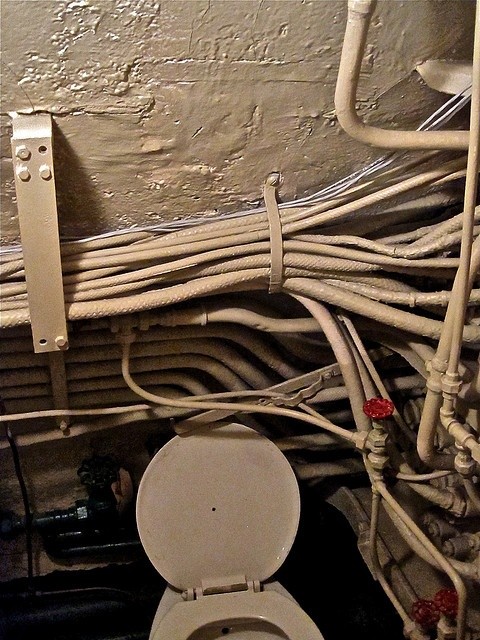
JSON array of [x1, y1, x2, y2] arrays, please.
[[134, 420, 326, 638]]
